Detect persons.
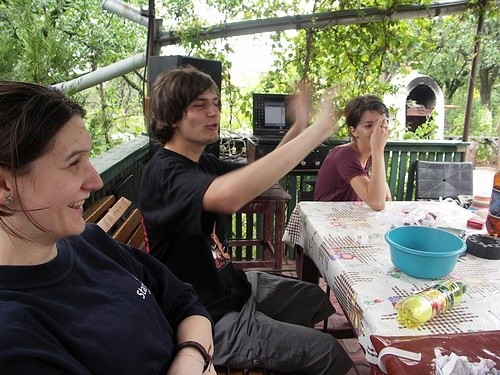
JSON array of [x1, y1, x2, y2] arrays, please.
[[0, 81, 219, 375], [145, 66, 392, 375]]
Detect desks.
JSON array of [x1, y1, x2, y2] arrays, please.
[[223, 180, 291, 271], [247, 138, 328, 175], [282, 201, 500, 375]]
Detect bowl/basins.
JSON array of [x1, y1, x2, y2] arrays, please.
[[383, 225, 467, 279]]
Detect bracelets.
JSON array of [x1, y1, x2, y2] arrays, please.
[[175, 354, 206, 367]]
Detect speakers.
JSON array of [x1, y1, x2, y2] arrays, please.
[[149, 55, 222, 157]]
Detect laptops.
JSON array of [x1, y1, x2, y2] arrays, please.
[[252, 93, 296, 145]]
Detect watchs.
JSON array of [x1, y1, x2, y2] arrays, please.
[[178, 340, 211, 373]]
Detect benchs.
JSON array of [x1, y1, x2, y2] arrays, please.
[[82, 194, 147, 252]]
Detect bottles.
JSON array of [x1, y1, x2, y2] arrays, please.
[[485, 170, 500, 238], [395, 279, 467, 329]]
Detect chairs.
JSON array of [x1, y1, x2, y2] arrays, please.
[[416, 161, 474, 200]]
